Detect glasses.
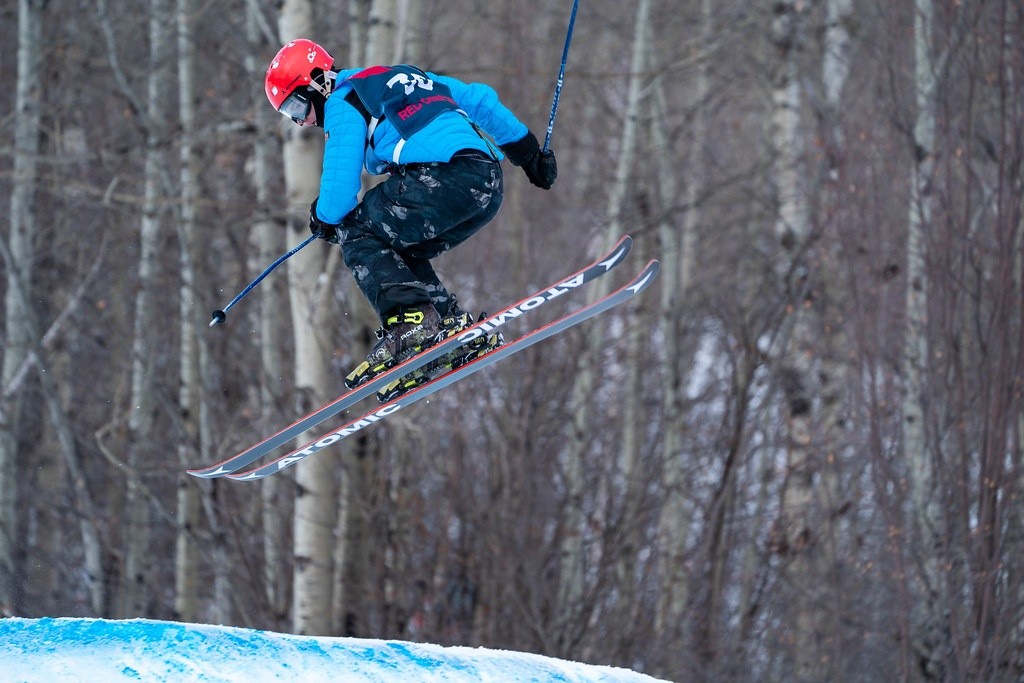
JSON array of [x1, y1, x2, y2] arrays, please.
[[278, 93, 311, 124]]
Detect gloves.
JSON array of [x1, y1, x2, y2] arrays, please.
[[309, 197, 340, 244], [522, 149, 557, 190]]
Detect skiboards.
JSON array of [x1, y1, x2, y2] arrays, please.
[[180, 232, 663, 484]]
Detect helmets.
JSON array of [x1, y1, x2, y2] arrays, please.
[[264, 38, 334, 111]]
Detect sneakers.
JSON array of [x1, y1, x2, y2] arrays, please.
[[402, 313, 480, 381], [366, 302, 446, 372]]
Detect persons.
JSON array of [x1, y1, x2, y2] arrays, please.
[[265, 39, 558, 403]]
